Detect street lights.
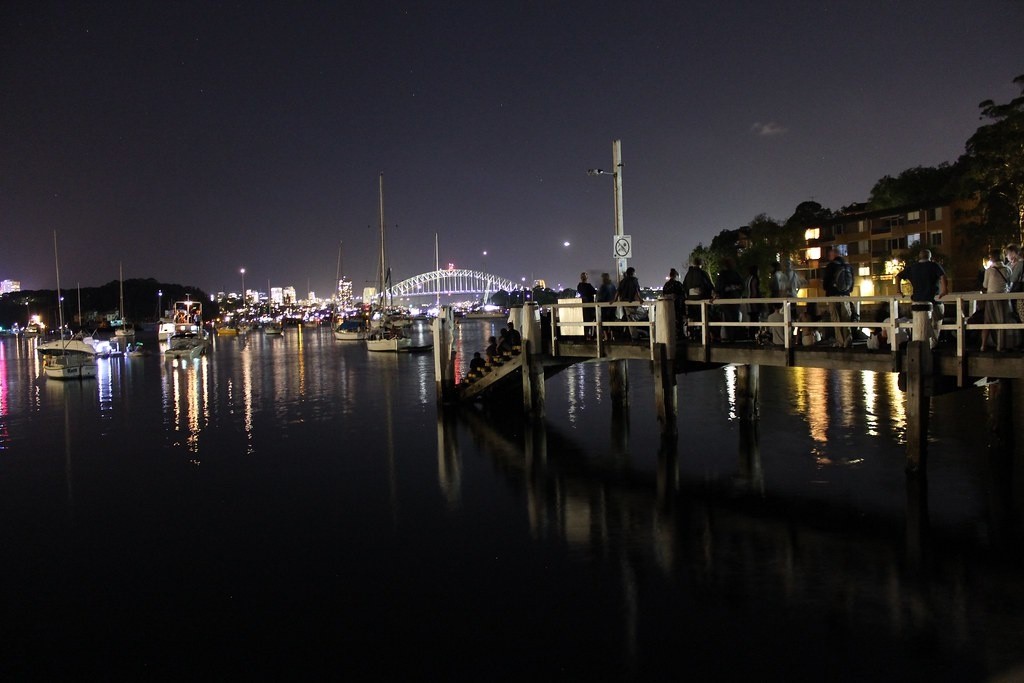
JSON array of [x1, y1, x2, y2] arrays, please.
[[239, 269, 246, 311], [158, 289, 163, 323], [587, 169, 619, 298]]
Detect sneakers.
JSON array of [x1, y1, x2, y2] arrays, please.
[[834, 337, 852, 349]]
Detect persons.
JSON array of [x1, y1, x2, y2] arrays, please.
[[662, 268, 686, 326], [469, 322, 520, 372], [977, 244, 1024, 353], [822, 248, 854, 347], [682, 255, 717, 340], [596, 273, 617, 341], [895, 249, 950, 349], [713, 260, 824, 345], [609, 267, 644, 341], [577, 271, 598, 341]]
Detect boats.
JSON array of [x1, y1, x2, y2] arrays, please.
[[215, 318, 284, 337], [173, 293, 203, 337], [165, 337, 210, 362]]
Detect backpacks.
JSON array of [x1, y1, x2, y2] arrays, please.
[[832, 260, 853, 293]]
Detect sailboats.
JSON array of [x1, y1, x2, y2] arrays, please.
[[44, 379, 98, 495], [114, 261, 135, 337], [330, 238, 375, 342], [35, 230, 99, 379], [365, 172, 434, 352]]
